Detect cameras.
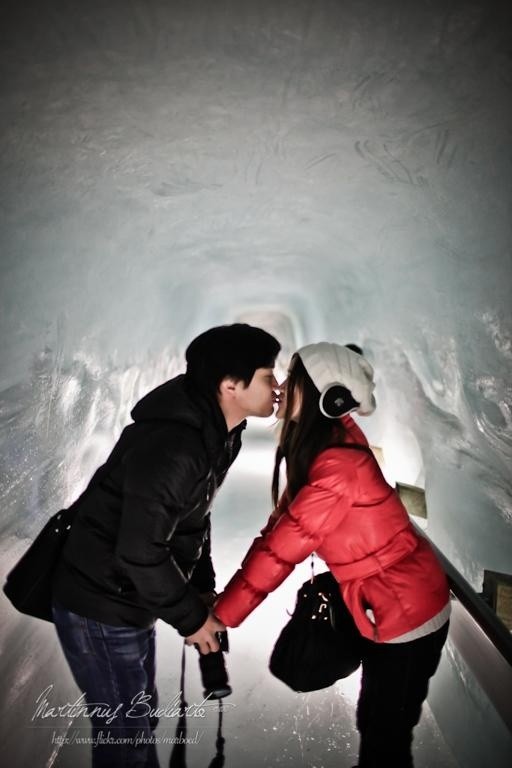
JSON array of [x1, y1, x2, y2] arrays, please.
[[185, 631, 232, 700]]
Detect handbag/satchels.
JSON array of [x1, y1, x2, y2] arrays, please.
[[267, 550, 361, 694], [2, 508, 71, 628]]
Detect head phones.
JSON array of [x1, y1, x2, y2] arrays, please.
[[319, 383, 360, 419]]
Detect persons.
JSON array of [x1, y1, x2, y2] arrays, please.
[[48, 320, 278, 768], [202, 340, 458, 766]]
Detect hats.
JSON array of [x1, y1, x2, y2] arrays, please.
[[296, 342, 377, 418]]
